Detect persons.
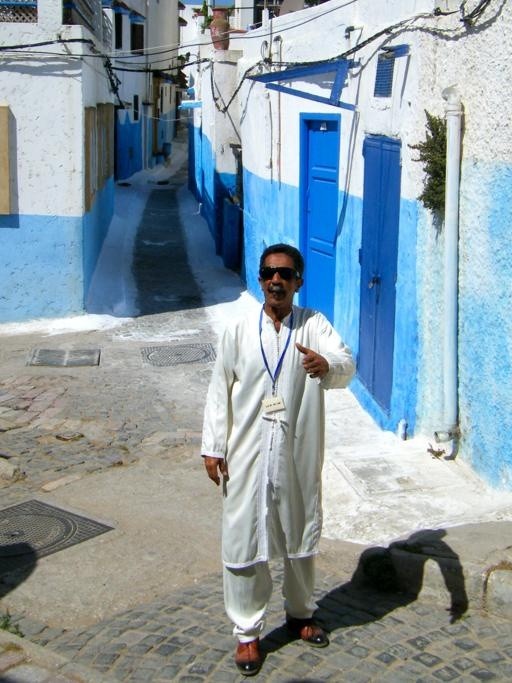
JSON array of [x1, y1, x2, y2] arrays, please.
[[198, 240, 358, 679]]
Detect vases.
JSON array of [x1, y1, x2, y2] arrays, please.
[[210, 8, 230, 50]]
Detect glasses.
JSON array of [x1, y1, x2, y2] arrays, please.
[[259, 266, 301, 281]]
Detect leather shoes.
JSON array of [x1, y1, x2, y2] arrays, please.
[[234, 636, 262, 675], [286, 614, 329, 648]]
